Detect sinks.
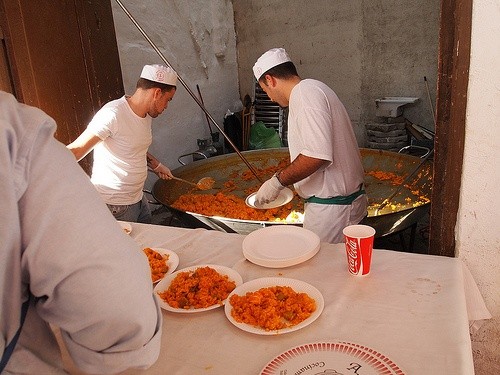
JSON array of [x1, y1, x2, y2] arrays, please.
[[375, 97, 419, 117]]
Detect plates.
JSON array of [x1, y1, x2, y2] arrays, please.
[[118, 222, 132, 235], [154, 264, 243, 313], [225, 277, 324, 335], [244, 187, 293, 209], [242, 224, 321, 269], [142, 248, 179, 284], [259, 341, 406, 375]]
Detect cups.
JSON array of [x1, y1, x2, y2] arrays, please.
[[342, 225, 376, 276]]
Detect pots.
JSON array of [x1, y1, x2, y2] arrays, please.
[[142, 145, 433, 239]]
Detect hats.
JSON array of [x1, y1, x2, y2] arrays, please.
[[253, 47, 292, 81], [140, 64, 177, 87]]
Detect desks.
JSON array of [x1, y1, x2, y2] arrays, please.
[[127, 223, 492, 375]]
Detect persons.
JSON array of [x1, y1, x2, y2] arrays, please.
[[252, 47, 370, 245], [66, 64, 177, 224], [0, 91, 163, 375]]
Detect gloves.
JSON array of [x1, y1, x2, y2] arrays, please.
[[151, 164, 173, 180], [255, 172, 286, 205]]
